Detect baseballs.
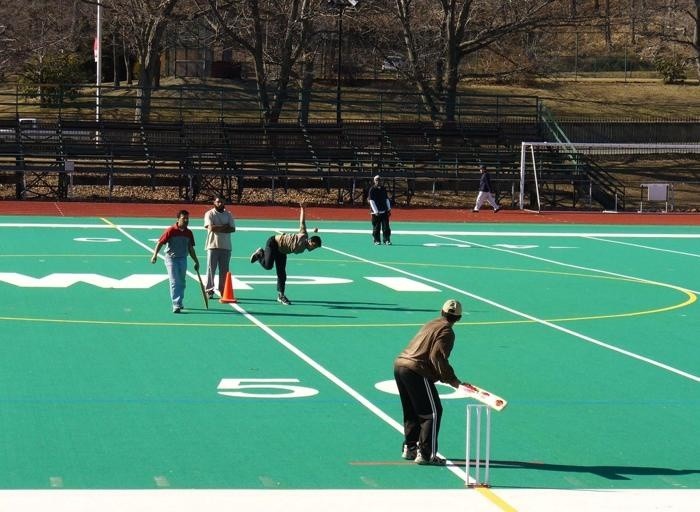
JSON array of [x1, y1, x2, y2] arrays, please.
[[314, 228, 319, 234]]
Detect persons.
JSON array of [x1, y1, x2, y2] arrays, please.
[[249, 203, 321, 305], [394, 300, 462, 465], [368, 175, 391, 245], [205, 195, 235, 298], [151, 211, 199, 312], [471, 164, 503, 212]]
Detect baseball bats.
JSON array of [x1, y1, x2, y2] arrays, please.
[[196, 269, 209, 309]]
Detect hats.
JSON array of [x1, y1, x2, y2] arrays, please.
[[494, 208, 500, 213], [442, 299, 462, 316], [373, 174, 383, 181], [478, 165, 486, 170]]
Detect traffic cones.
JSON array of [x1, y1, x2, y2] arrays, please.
[[221, 272, 236, 303]]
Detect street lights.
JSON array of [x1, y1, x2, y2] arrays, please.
[[327, 0, 352, 166]]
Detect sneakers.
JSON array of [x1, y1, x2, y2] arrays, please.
[[472, 210, 479, 213], [414, 449, 446, 466], [277, 294, 291, 305], [374, 241, 380, 245], [173, 305, 180, 313], [207, 291, 213, 299], [401, 444, 419, 460], [384, 240, 391, 245], [250, 248, 264, 264]]
